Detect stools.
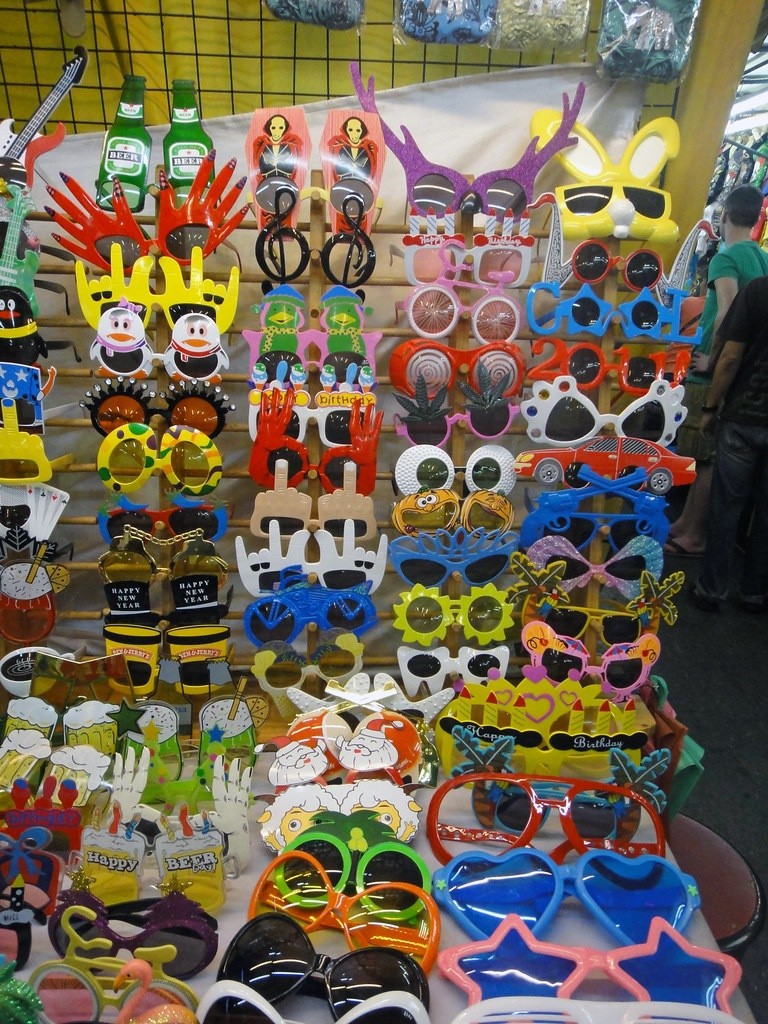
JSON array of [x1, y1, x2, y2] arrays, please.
[[665, 812, 765, 964]]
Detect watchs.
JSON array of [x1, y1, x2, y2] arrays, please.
[[701, 400, 719, 413]]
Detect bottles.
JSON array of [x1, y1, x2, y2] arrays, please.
[[98, 74, 153, 212], [162, 79, 215, 208]]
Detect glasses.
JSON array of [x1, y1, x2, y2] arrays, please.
[[7, 67, 721, 1023]]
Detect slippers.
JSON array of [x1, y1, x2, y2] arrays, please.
[[663, 534, 705, 558]]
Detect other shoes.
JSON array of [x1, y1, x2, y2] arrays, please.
[[733, 581, 767, 612], [689, 574, 731, 612]]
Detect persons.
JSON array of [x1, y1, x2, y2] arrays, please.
[[664, 187, 768, 557], [689, 276, 768, 612]]
